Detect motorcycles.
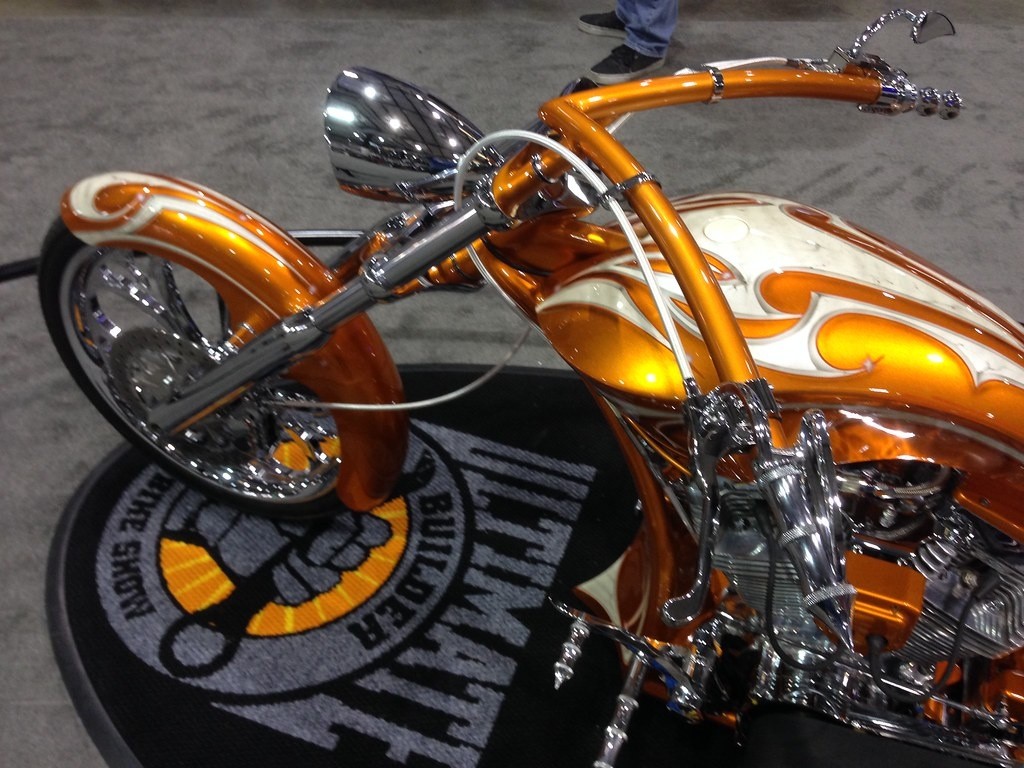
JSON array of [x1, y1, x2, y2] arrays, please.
[[36, 8, 1022, 767]]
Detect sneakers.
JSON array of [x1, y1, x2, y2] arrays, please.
[[590, 43, 665, 82], [578, 11, 628, 38]]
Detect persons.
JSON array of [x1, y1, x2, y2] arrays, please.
[[577, 1, 678, 87]]
